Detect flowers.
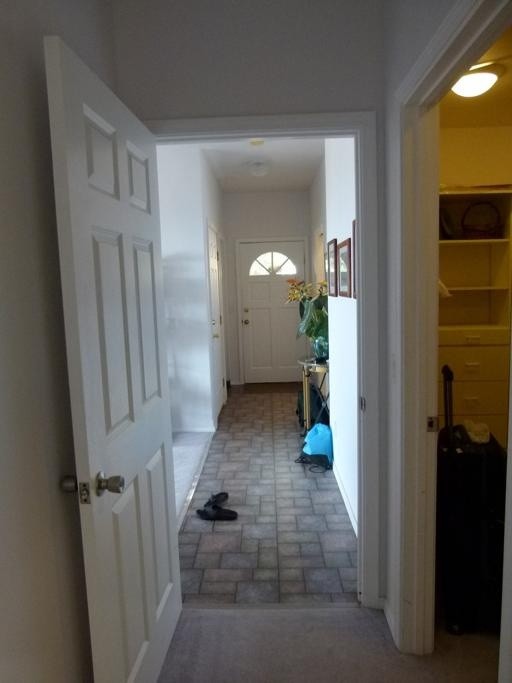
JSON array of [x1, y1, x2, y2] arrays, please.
[[284, 279, 329, 343]]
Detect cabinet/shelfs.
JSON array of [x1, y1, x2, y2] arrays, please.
[[437, 185, 512, 451]]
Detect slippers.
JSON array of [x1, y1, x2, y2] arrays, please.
[[197, 493, 237, 520]]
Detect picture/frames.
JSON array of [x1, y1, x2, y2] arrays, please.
[[327, 218, 358, 299]]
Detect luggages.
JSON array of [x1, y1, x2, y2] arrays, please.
[[436, 364, 506, 638]]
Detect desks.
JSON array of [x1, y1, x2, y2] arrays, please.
[[296, 359, 330, 436]]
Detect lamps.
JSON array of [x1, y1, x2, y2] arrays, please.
[[450, 63, 504, 99], [247, 161, 269, 177]]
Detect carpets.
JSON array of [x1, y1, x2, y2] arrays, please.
[[155, 606, 500, 683]]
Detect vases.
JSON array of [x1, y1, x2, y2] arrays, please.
[[313, 336, 329, 364]]
